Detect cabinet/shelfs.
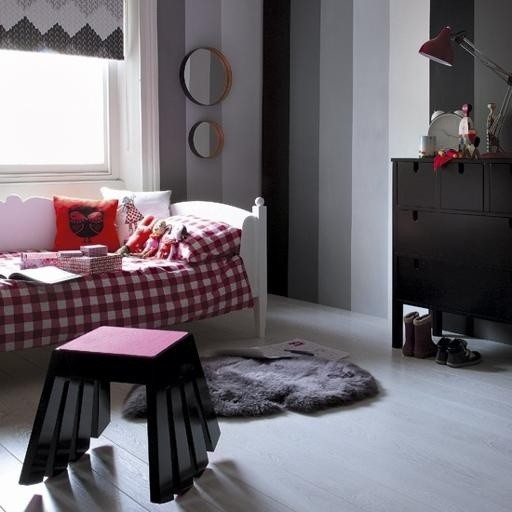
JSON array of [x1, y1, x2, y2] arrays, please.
[[392, 158, 512, 348]]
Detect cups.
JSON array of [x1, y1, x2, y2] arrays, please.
[[420, 136, 435, 157]]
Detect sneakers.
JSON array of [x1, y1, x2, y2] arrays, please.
[[434, 338, 451, 365], [446, 339, 481, 367]]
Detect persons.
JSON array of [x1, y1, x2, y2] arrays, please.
[[120, 195, 144, 236]]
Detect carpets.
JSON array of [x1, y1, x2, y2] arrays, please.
[[122, 354, 380, 419]]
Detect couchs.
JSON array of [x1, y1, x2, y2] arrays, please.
[[0, 195, 268, 351]]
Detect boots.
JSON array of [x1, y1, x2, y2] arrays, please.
[[402, 310, 420, 356], [413, 312, 440, 359]]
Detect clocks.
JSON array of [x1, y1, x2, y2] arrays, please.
[[426, 109, 464, 154]]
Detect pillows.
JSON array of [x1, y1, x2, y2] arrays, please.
[[156, 215, 241, 265], [100, 187, 171, 247], [52, 196, 120, 252]]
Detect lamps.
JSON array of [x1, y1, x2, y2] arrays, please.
[[418, 26, 512, 158]]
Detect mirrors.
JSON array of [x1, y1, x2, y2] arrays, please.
[[179, 46, 232, 106], [188, 120, 224, 159]]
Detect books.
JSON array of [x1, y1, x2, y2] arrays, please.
[[0, 265, 84, 286]]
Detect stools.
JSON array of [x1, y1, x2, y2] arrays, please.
[[18, 326, 220, 503]]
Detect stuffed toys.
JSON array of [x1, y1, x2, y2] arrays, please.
[[118, 215, 188, 260]]
